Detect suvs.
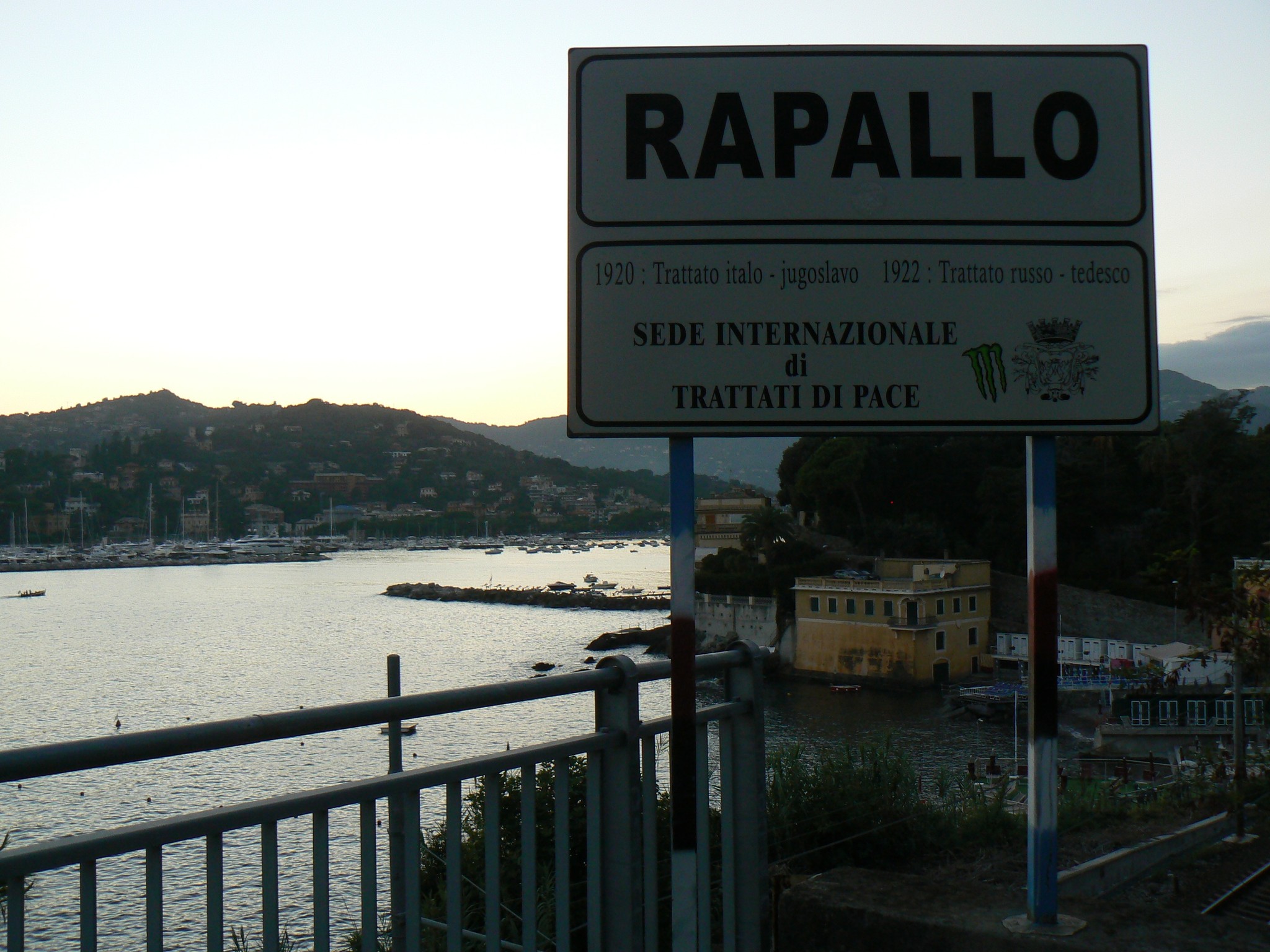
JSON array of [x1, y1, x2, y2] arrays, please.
[[834, 569, 881, 581]]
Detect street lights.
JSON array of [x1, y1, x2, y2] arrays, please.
[[1172, 580, 1179, 643]]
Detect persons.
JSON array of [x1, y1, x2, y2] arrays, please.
[[23, 591, 25, 594], [26, 590, 28, 594], [1065, 666, 1121, 686], [29, 589, 31, 594]]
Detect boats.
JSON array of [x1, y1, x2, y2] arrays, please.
[[830, 684, 861, 692], [0, 480, 671, 565], [17, 589, 47, 597]]
